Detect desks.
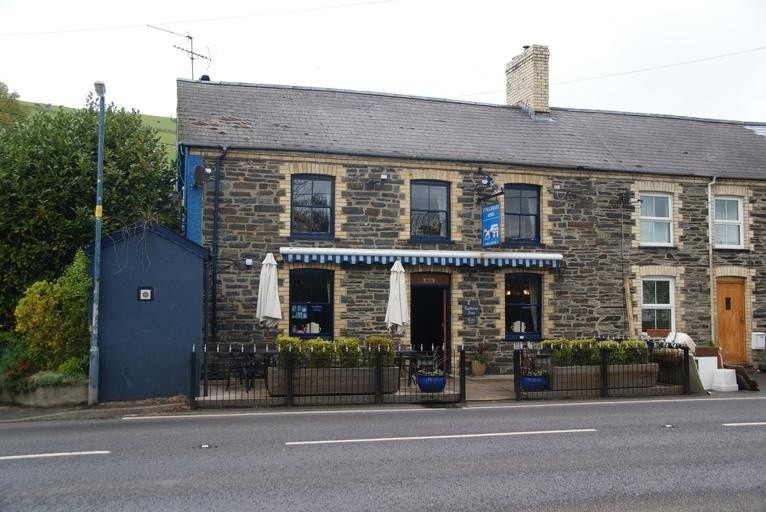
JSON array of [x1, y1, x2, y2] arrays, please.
[[399, 349, 440, 387], [249, 352, 281, 391]]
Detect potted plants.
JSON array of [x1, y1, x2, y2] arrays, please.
[[416, 369, 448, 392], [520, 367, 548, 392], [470, 345, 489, 376]]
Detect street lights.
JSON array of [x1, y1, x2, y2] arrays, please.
[[85, 82, 109, 412]]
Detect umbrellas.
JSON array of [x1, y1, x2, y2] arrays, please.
[[256, 253, 282, 345], [385, 260, 409, 332]]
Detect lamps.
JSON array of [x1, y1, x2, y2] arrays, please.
[[245, 254, 254, 266], [552, 184, 576, 200], [365, 174, 388, 192]]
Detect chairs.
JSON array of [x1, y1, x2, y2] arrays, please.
[[226, 342, 255, 392]]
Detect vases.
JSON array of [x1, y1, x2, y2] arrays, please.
[[645, 328, 669, 337]]
